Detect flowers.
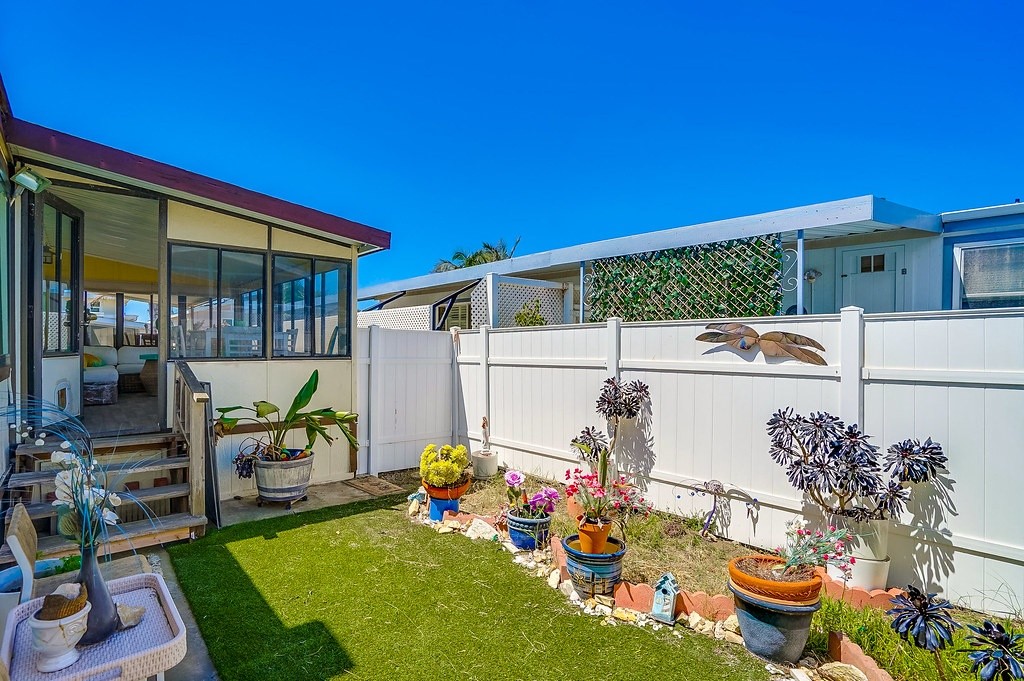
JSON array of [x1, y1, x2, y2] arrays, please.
[[0, 390, 187, 589], [774, 523, 876, 581], [420, 444, 469, 487], [504, 470, 560, 519], [564, 469, 654, 541]]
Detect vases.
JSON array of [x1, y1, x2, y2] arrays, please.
[[507, 508, 551, 551], [727, 581, 821, 664], [576, 514, 612, 555], [561, 534, 628, 599], [727, 555, 823, 601], [421, 478, 471, 500], [74, 544, 119, 646]]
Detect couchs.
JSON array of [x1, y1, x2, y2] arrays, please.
[[84, 346, 119, 405], [116, 346, 180, 392]]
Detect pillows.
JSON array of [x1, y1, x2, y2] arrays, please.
[[83, 353, 107, 371]]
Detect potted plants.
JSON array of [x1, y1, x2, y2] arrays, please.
[[564, 376, 648, 515], [766, 407, 947, 592], [208, 369, 360, 502]]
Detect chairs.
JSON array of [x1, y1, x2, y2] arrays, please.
[[180, 325, 298, 358], [6, 503, 151, 605]]
